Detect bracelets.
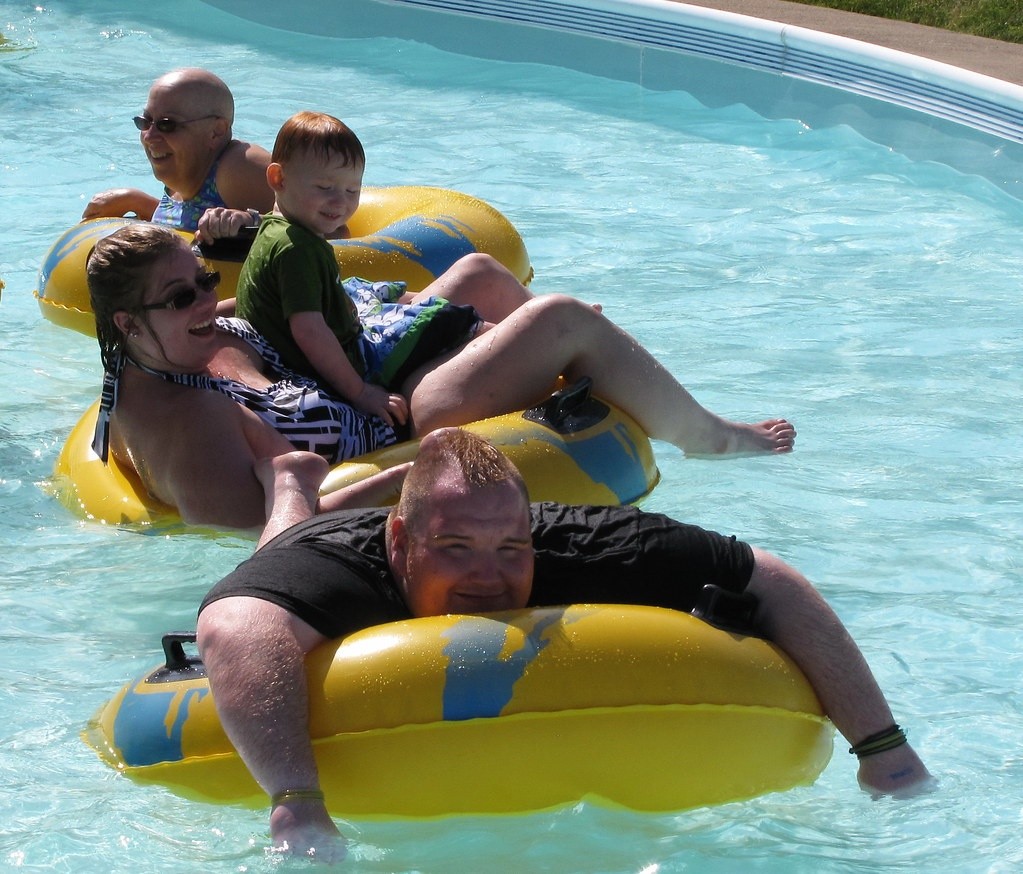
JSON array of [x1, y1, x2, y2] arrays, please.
[[271, 790, 325, 803], [849, 724, 907, 758]]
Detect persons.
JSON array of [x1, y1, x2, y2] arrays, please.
[[229, 111, 601, 431], [196, 427, 931, 847], [86, 225, 796, 529], [78, 68, 352, 241]]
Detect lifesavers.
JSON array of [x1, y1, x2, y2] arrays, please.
[[30, 186, 535, 336], [84, 631, 835, 821], [54, 389, 663, 543]]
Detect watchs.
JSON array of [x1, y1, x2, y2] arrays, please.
[[245, 208, 260, 226]]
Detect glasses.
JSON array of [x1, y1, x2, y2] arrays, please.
[[132, 115, 218, 133], [127, 271, 221, 311]]
